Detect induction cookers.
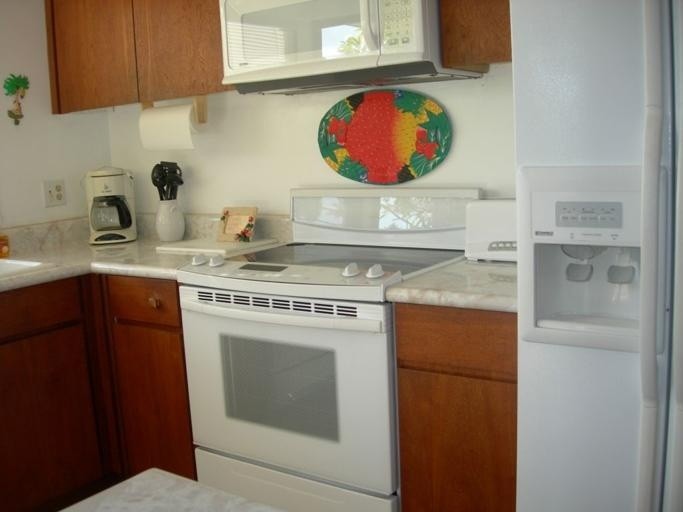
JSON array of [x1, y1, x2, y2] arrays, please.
[[178, 242, 464, 303]]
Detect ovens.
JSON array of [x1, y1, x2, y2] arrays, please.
[[178, 284, 398, 512]]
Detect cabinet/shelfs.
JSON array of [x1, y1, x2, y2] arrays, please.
[[100, 273, 195, 480], [439, 0, 511, 73], [46, 0, 226, 112], [389, 302, 516, 512], [0, 276, 97, 511]]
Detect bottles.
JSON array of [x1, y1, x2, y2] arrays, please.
[[156, 200, 185, 241]]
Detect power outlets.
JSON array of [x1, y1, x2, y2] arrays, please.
[[42, 180, 65, 207]]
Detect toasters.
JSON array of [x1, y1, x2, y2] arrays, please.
[[464, 199, 516, 262]]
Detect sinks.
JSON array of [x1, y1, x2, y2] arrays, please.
[[0, 259, 59, 281]]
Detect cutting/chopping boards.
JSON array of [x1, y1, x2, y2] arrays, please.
[[156, 237, 279, 253]]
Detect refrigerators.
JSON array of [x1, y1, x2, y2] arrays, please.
[[510, 1, 683, 511]]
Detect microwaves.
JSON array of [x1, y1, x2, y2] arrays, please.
[[217, 0, 442, 85]]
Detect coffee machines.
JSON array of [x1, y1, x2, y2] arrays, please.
[[88, 168, 138, 244]]
